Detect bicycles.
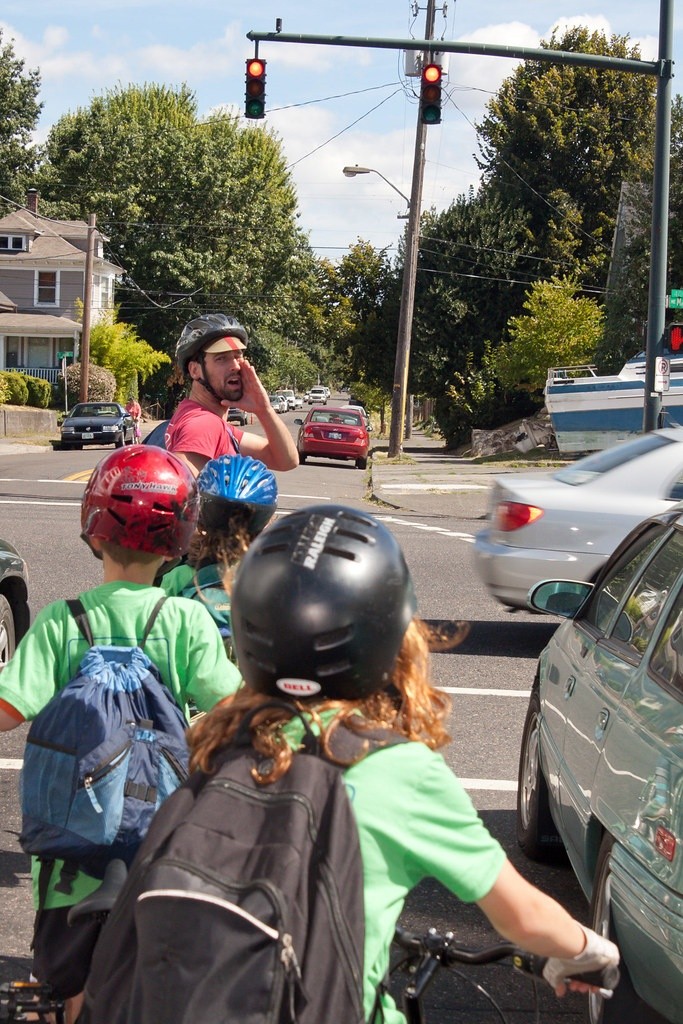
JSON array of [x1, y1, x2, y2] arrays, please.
[[388, 924, 620, 1024], [0, 856, 127, 1024]]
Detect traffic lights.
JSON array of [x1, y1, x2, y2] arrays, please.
[[245, 57, 269, 119], [665, 322, 683, 354], [422, 64, 443, 124]]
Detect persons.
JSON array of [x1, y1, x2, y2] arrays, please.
[[164, 313, 300, 479], [0, 445, 248, 1024], [125, 397, 142, 443], [185, 504, 620, 1024], [160, 454, 279, 715]]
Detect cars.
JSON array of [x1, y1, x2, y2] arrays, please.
[[295, 406, 371, 471], [517, 502, 683, 1024], [337, 404, 369, 426], [472, 426, 683, 614], [268, 384, 331, 414], [227, 406, 248, 426], [60, 401, 136, 451]]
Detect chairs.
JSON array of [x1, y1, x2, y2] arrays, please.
[[316, 416, 328, 423], [343, 419, 357, 425]]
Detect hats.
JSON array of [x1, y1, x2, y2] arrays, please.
[[202, 336, 248, 354]]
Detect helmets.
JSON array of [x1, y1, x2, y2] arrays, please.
[[194, 453, 278, 525], [80, 445, 197, 563], [175, 314, 248, 378], [231, 503, 417, 697]]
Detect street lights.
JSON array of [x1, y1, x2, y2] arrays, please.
[[342, 164, 425, 458]]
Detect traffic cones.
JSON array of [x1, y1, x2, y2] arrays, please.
[[250, 413, 254, 424]]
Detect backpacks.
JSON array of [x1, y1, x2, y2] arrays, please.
[[18, 593, 193, 861], [73, 715, 414, 1024]]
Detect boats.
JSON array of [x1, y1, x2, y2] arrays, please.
[[542, 349, 683, 458]]
